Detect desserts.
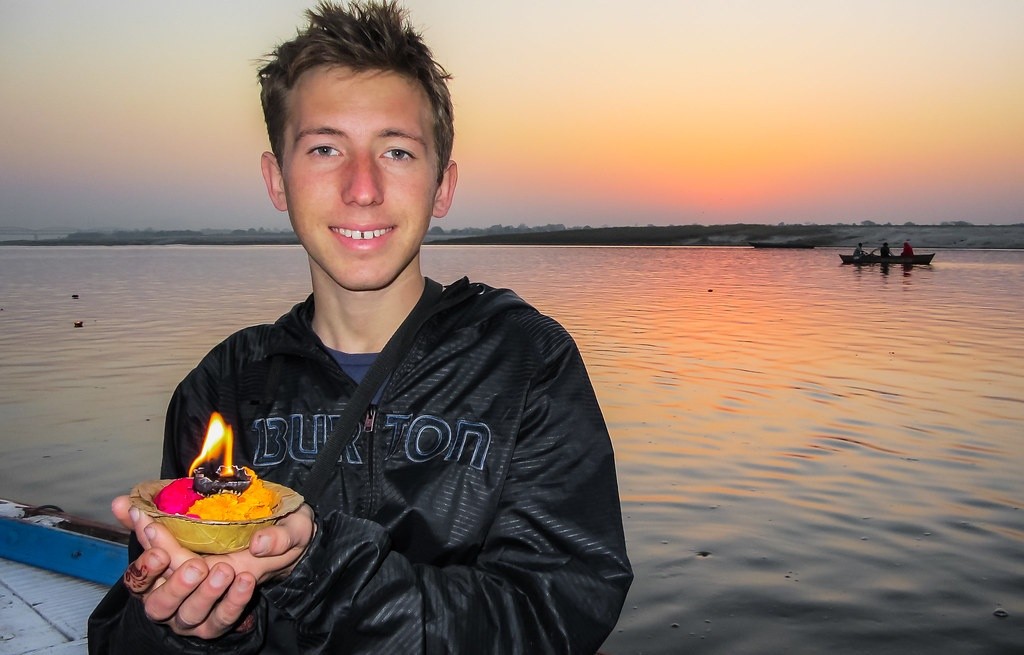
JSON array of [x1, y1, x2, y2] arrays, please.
[[131, 464, 305, 555]]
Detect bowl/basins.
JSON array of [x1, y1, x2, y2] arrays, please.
[[128, 479, 305, 554]]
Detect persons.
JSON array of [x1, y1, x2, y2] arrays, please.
[[880, 242, 895, 257], [87, 0, 634, 655], [901, 241, 913, 256], [853, 242, 865, 256]]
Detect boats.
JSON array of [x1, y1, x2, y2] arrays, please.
[[839, 252, 936, 265]]
